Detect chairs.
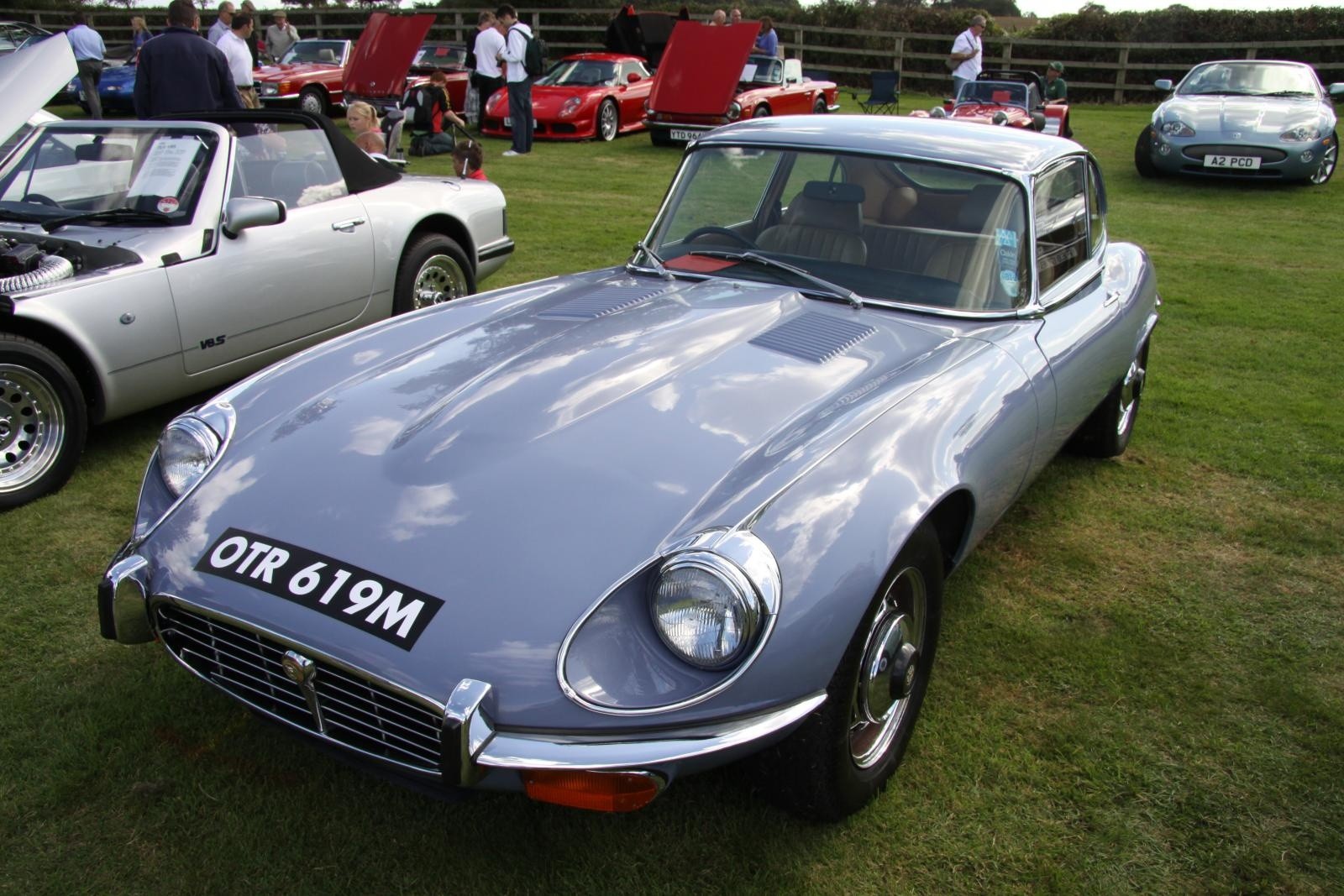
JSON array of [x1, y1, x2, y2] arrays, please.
[[319, 49, 339, 64], [582, 67, 603, 83], [857, 68, 900, 116], [803, 70, 831, 83], [273, 159, 329, 210], [751, 191, 868, 265], [992, 90, 1011, 103], [931, 184, 1026, 311]]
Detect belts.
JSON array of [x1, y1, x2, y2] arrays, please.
[[238, 85, 253, 90]]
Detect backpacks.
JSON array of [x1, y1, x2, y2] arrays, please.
[[507, 28, 549, 76]]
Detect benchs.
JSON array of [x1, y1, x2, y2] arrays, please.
[[858, 223, 1074, 280], [229, 160, 346, 206]]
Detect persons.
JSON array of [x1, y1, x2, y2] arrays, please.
[[1039, 60, 1073, 139], [208, 0, 235, 46], [132, 0, 264, 155], [265, 11, 300, 64], [493, 5, 535, 155], [355, 131, 386, 156], [236, 0, 260, 67], [751, 16, 779, 77], [216, 11, 261, 108], [67, 8, 106, 120], [449, 139, 489, 181], [345, 99, 383, 133], [402, 69, 465, 157], [726, 9, 741, 26], [708, 10, 726, 26], [465, 3, 507, 139], [950, 15, 987, 98], [130, 17, 154, 55]]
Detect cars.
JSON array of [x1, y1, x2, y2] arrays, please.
[[0, 32, 138, 212], [252, 38, 351, 119], [0, 21, 67, 107]]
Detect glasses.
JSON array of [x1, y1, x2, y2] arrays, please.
[[226, 11, 236, 16], [978, 24, 986, 29]]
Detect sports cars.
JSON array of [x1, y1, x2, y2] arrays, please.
[[96, 113, 1167, 827], [337, 13, 510, 117], [65, 44, 265, 116], [480, 52, 657, 143], [909, 69, 1069, 139], [643, 19, 840, 158], [1134, 60, 1344, 185], [0, 118, 516, 513]]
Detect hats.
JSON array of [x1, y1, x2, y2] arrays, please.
[[273, 10, 287, 17], [1049, 61, 1064, 73]]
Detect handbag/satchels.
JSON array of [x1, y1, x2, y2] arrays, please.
[[944, 55, 960, 70]]
